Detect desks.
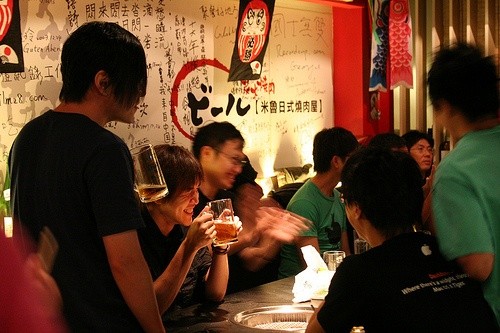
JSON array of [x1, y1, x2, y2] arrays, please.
[[162, 277, 332, 333]]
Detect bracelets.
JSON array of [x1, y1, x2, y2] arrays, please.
[[211, 243, 231, 255]]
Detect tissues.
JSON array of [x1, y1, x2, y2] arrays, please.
[[292, 244, 336, 301]]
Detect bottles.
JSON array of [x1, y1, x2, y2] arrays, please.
[[0, 161, 13, 237]]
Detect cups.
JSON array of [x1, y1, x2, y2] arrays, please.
[[323, 251, 345, 271], [353, 230, 371, 254], [129, 144, 169, 203], [202, 198, 238, 246]]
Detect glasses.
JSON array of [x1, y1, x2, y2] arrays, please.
[[410, 146, 434, 153], [340, 193, 356, 204], [211, 147, 246, 167]]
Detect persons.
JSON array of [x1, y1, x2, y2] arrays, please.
[[177, 121, 312, 295], [279, 126, 436, 281], [7, 21, 166, 333], [304, 144, 500, 333], [431, 40, 500, 324], [135, 144, 244, 317]]
